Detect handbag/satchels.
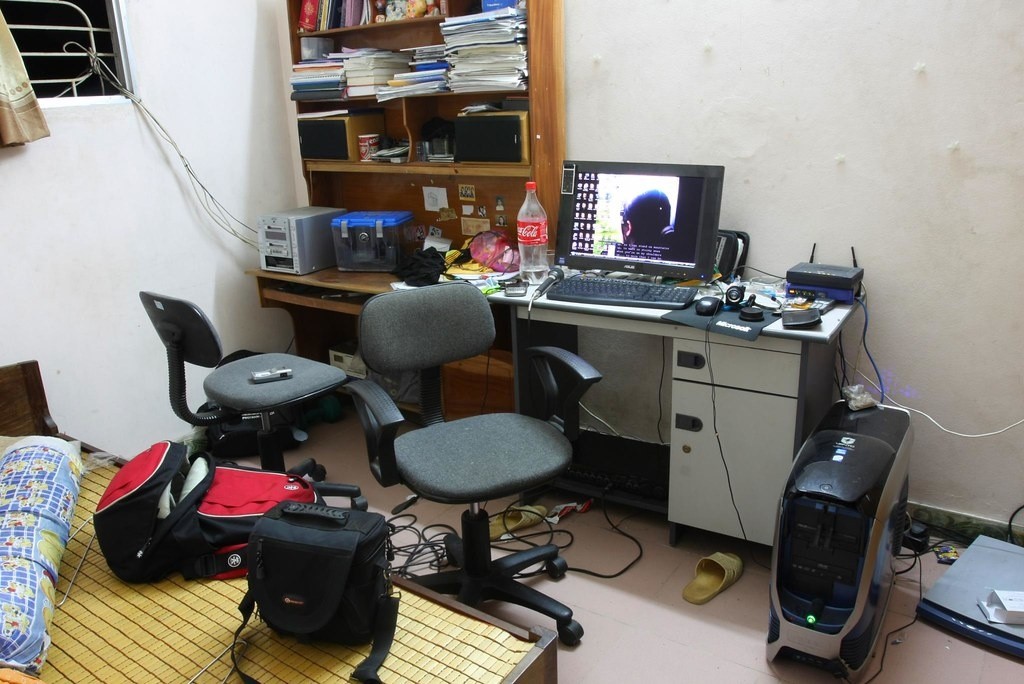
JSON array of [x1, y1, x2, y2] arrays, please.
[[470, 230, 520, 272], [390, 246, 451, 287], [231, 500, 402, 684]]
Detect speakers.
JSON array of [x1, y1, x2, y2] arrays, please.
[[456, 111, 531, 166], [299, 113, 384, 162]]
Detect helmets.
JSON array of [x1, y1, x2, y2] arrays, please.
[[623, 191, 671, 226]]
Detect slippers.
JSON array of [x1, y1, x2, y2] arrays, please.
[[489, 505, 547, 541], [683, 552, 742, 605]]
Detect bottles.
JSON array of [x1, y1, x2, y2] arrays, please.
[[517, 182, 550, 284]]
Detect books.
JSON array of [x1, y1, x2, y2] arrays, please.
[[438, 8, 528, 92], [290, 45, 448, 102], [296, 106, 375, 117], [294, 1, 375, 31]]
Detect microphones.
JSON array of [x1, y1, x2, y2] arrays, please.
[[534, 268, 564, 298]]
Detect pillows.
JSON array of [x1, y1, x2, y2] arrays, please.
[[0, 436, 81, 684]]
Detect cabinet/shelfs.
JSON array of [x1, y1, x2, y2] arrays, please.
[[285, 0, 567, 262]]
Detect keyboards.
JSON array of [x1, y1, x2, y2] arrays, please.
[[547, 276, 698, 309]]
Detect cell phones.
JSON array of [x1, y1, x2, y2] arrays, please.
[[251, 367, 293, 382], [506, 282, 527, 297], [811, 298, 836, 314]]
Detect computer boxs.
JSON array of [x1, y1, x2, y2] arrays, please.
[[765, 400, 912, 684]]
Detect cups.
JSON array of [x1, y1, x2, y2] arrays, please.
[[358, 133, 378, 162]]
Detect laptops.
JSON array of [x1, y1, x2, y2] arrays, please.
[[915, 535, 1024, 658]]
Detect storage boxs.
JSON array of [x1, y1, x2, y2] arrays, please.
[[331, 210, 414, 272], [481, 0, 519, 13]]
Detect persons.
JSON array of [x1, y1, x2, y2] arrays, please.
[[496, 198, 504, 210], [620, 189, 675, 244], [496, 216, 506, 226]]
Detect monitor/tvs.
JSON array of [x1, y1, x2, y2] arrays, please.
[[554, 160, 725, 282]]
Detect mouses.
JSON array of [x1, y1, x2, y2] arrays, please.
[[696, 297, 724, 316]]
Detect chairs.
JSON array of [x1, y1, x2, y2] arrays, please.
[[140, 290, 369, 511], [342, 281, 602, 644]]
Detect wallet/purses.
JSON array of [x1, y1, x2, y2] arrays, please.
[[782, 308, 823, 329]]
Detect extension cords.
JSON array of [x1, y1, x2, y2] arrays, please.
[[902, 524, 929, 551]]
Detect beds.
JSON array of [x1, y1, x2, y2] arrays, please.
[[0, 361, 560, 684]]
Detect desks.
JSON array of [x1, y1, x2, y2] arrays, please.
[[486, 276, 866, 548], [246, 251, 514, 421]]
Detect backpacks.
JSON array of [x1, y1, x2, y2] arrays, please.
[[93, 440, 327, 584]]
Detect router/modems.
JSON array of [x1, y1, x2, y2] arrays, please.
[[786, 243, 865, 290]]
